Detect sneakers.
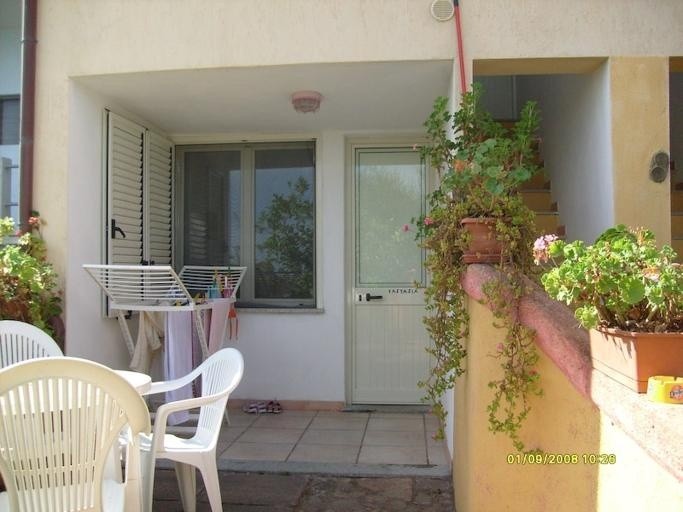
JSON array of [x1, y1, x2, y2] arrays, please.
[[241, 400, 281, 414]]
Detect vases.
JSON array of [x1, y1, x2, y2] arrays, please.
[[1, 291, 26, 320], [588, 327, 683, 395]]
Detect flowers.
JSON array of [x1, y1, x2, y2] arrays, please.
[[0, 210, 62, 336], [531, 224, 682, 333]]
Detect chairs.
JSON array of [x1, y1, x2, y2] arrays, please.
[[1, 356, 152, 511], [120, 346, 245, 512], [0, 320, 63, 368]]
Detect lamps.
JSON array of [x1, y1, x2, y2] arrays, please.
[[291, 90, 322, 113]]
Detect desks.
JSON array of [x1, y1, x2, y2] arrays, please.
[[1, 370, 152, 418]]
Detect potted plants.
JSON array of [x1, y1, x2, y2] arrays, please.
[[443, 138, 530, 265]]
